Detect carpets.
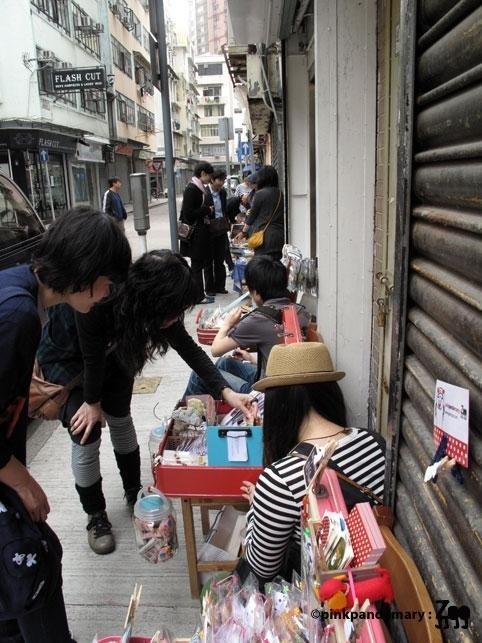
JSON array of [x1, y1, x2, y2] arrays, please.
[[133, 377, 161, 393]]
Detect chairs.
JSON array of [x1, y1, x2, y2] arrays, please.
[[374, 502, 446, 643]]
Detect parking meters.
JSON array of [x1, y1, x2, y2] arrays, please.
[[130, 173, 150, 255]]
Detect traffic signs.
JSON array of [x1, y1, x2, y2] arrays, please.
[[53, 68, 104, 91]]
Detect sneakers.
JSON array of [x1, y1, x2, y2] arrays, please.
[[122, 486, 145, 516], [86, 510, 115, 555]]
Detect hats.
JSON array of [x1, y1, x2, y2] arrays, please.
[[252, 342, 345, 392]]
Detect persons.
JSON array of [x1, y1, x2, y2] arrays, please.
[[1, 162, 386, 643]]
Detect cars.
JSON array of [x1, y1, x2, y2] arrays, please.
[[0, 172, 49, 272], [223, 176, 241, 194]]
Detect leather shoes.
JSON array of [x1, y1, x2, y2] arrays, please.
[[199, 287, 228, 304]]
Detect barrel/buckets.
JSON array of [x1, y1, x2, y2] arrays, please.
[[147, 416, 168, 481], [131, 487, 178, 563]]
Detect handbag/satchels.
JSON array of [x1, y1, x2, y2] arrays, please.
[[176, 221, 195, 242], [27, 353, 71, 422], [212, 216, 231, 236], [0, 510, 58, 624], [248, 230, 265, 248]]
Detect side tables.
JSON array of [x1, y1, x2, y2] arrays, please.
[[180, 494, 251, 601]]
